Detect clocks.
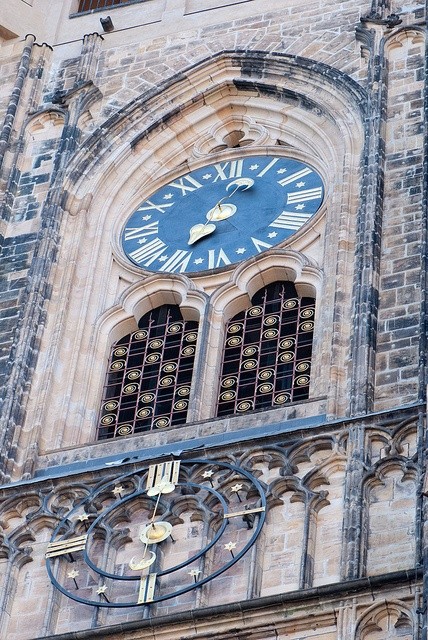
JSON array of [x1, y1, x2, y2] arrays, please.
[[41, 456, 270, 613], [106, 146, 331, 288]]
